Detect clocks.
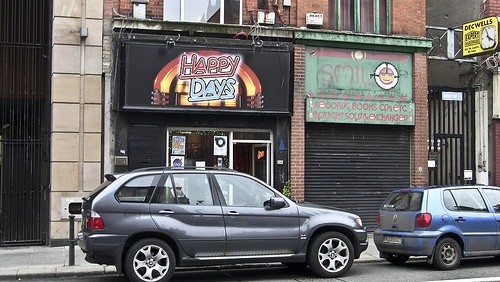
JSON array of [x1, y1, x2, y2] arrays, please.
[[480, 25, 496, 50]]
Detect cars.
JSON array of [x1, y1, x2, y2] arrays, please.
[[373, 185, 500, 270], [77, 166, 369, 282]]
